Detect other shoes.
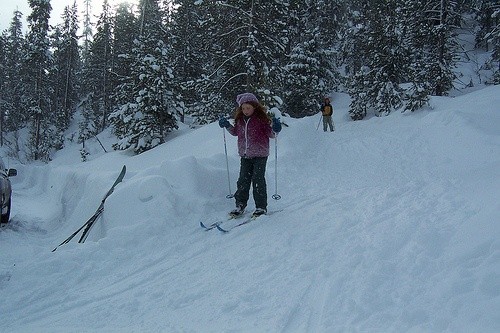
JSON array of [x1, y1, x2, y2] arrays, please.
[[331, 130, 334, 131]]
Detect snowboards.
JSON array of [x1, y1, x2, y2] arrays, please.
[[77, 164, 128, 244]]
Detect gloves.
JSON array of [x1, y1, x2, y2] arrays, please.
[[271, 117, 282, 133], [219, 118, 230, 129]]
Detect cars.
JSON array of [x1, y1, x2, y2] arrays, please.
[[0, 156, 18, 225]]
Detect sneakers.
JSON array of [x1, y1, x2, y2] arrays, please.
[[252, 207, 267, 217], [228, 208, 244, 219]]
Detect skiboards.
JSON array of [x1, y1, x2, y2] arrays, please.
[[201, 202, 284, 233]]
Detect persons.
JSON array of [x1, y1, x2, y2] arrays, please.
[[219, 93, 281, 216], [320, 98, 334, 132]]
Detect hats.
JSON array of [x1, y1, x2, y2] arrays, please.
[[236, 93, 259, 108], [325, 98, 329, 101]]
